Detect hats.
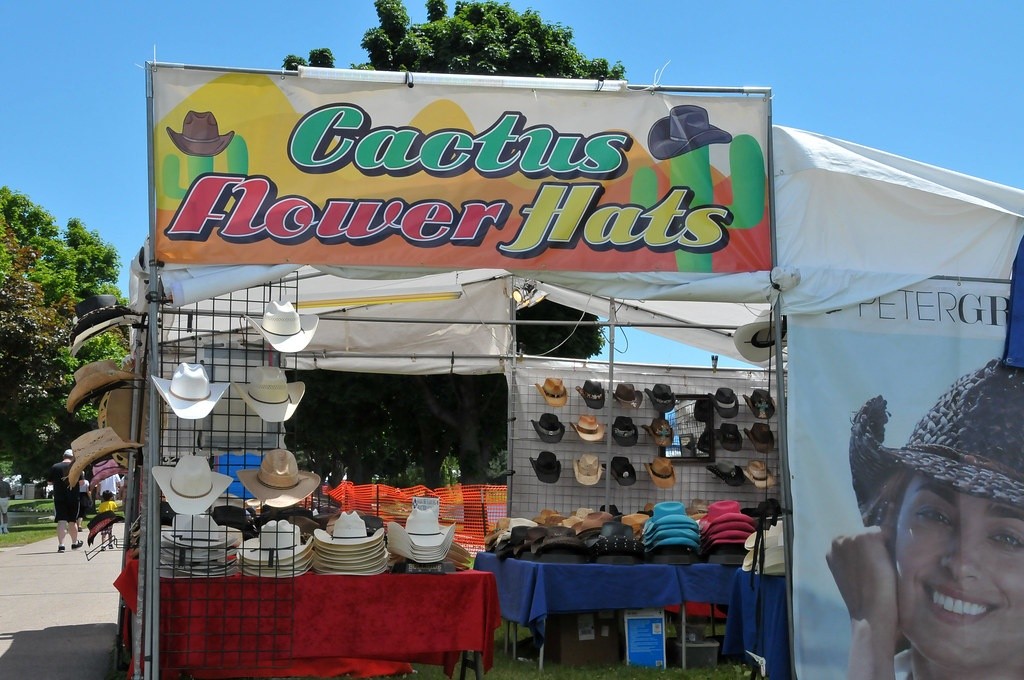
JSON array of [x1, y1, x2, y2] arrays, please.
[[244, 301, 320, 353], [236, 449, 321, 508], [68, 294, 143, 356], [150, 361, 232, 419], [151, 454, 232, 516], [86, 511, 126, 545], [529, 377, 678, 489], [64, 449, 73, 456], [705, 387, 780, 489], [483, 497, 793, 555], [849, 358, 1024, 527], [238, 367, 307, 423], [733, 310, 787, 362], [68, 360, 146, 491], [157, 506, 474, 576]]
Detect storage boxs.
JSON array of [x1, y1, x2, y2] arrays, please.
[[546, 608, 620, 667], [672, 636, 720, 667], [622, 608, 666, 670]]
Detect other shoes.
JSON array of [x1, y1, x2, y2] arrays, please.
[[72, 540, 84, 551], [78, 527, 82, 532], [0, 530, 9, 534], [58, 545, 66, 553], [101, 543, 114, 551]]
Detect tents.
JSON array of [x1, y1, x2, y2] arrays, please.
[[110, 74, 1024, 680]]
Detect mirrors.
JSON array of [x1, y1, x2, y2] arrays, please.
[[660, 394, 716, 464], [679, 434, 696, 457]]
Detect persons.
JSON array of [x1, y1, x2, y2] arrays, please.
[[47, 448, 91, 553], [825, 358, 1024, 680], [0, 474, 12, 535], [98, 474, 125, 551]]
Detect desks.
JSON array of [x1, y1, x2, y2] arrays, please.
[[113, 548, 503, 680], [471, 553, 786, 680]]
